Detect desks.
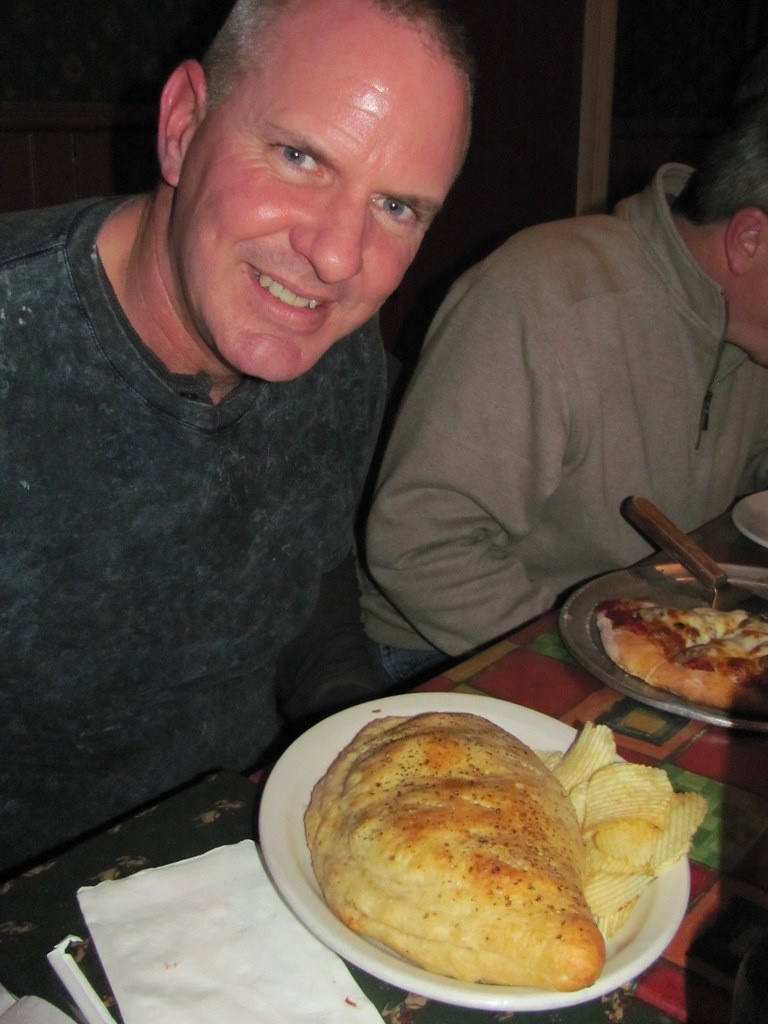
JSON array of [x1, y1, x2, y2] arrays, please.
[[0, 512, 768, 1024]]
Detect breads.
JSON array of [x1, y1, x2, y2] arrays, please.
[[305, 711, 606, 993]]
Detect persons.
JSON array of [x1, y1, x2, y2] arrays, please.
[[0, 0, 475, 885], [357, 97, 768, 695]]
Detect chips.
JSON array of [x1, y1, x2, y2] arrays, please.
[[533, 718, 709, 941]]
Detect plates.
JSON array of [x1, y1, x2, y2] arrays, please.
[[257, 690, 692, 1012], [559, 560, 768, 732], [731, 490, 768, 549]]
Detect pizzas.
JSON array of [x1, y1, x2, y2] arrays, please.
[[595, 596, 768, 716]]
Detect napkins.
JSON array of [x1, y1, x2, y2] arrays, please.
[[76, 838, 386, 1024]]
